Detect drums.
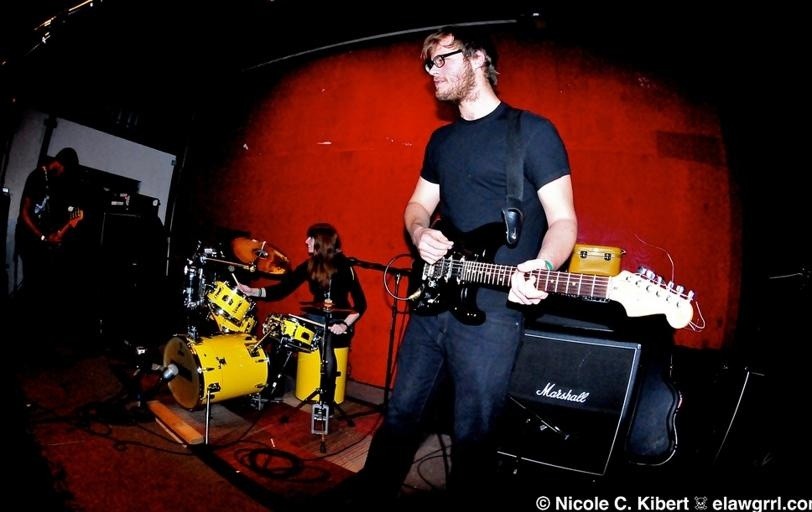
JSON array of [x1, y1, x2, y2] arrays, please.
[[207, 306, 256, 334], [164, 332, 270, 410], [206, 281, 256, 325], [263, 314, 316, 353]]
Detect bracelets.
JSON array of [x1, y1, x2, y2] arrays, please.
[[39, 232, 48, 242], [341, 321, 350, 328], [257, 286, 263, 298], [540, 256, 554, 272]]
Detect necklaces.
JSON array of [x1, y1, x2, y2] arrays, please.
[[42, 165, 52, 191]]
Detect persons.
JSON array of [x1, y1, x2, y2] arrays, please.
[[0, 146, 88, 342], [327, 24, 582, 510], [235, 221, 368, 421]]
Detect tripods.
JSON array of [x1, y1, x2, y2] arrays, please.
[[279, 269, 354, 453]]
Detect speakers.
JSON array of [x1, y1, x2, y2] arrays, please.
[[493, 330, 647, 480]]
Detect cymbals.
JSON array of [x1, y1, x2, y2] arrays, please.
[[207, 245, 224, 273], [232, 237, 290, 275], [301, 302, 356, 312]]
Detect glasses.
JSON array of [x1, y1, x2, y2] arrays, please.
[[424, 49, 462, 73]]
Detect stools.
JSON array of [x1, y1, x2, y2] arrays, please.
[[295, 345, 348, 407]]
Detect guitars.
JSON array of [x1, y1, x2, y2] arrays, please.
[[54, 208, 85, 242], [407, 218, 695, 330]]
